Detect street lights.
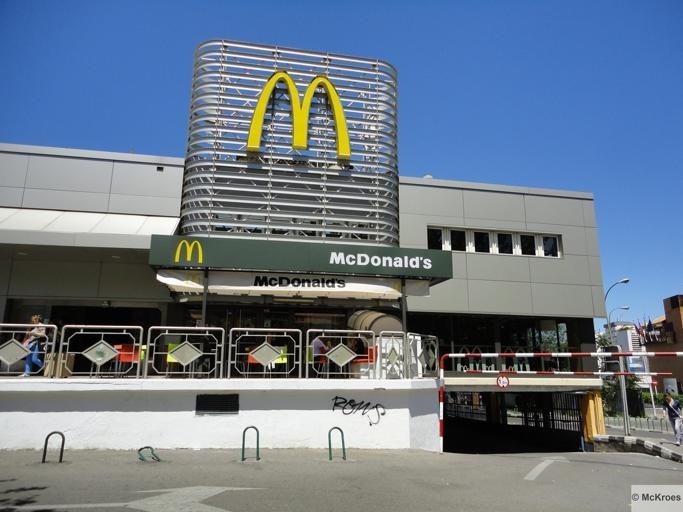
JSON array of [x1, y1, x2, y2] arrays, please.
[[604, 278, 629, 344]]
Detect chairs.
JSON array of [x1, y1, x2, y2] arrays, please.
[[89, 339, 378, 379]]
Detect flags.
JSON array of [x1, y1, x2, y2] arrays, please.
[[633, 320, 654, 337]]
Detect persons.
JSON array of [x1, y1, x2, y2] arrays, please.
[[309, 335, 329, 361], [20, 313, 47, 379], [662, 392, 683, 446], [346, 333, 365, 355]]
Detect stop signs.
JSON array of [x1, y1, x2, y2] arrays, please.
[[652, 380, 657, 385]]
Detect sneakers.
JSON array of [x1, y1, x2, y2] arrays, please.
[[20, 374, 30, 377], [676, 440, 681, 445]]
